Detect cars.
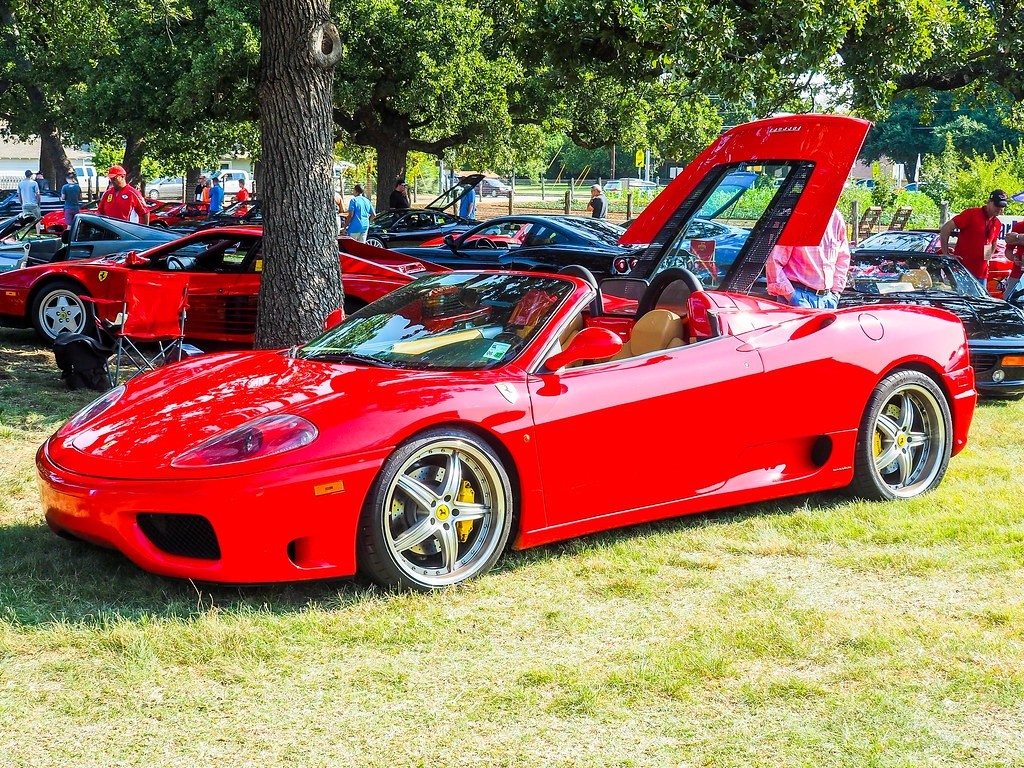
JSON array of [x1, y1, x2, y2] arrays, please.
[[386, 214, 694, 286], [143, 176, 187, 200], [604, 177, 656, 194], [831, 229, 1024, 402], [472, 179, 512, 198], [617, 171, 768, 286], [854, 177, 944, 193], [337, 174, 500, 250]]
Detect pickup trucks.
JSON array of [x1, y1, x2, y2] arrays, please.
[[202, 170, 257, 197], [66, 165, 110, 199]]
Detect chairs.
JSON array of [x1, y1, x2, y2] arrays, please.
[[608, 308, 687, 365], [80, 268, 191, 389], [49, 230, 71, 263], [689, 238, 718, 287], [531, 235, 548, 246], [898, 269, 932, 288], [517, 312, 583, 369]]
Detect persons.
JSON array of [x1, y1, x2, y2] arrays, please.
[[17, 169, 41, 240], [193, 175, 250, 218], [61, 172, 82, 231], [766, 207, 851, 309], [34, 171, 49, 193], [458, 176, 476, 220], [342, 184, 377, 244], [587, 184, 608, 219], [1003, 220, 1024, 300], [389, 179, 410, 208], [941, 189, 1009, 291], [97, 165, 150, 227], [334, 191, 345, 236]]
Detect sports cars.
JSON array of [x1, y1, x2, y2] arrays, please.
[[0, 185, 264, 272], [0, 223, 492, 353], [36, 112, 973, 595]]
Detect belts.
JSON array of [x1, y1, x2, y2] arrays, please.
[[790, 279, 833, 295]]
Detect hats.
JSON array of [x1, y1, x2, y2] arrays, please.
[[989, 189, 1008, 207], [106, 166, 126, 178], [25, 170, 33, 177], [65, 172, 74, 179]]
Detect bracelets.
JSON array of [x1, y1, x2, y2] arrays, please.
[[1016, 234, 1020, 242]]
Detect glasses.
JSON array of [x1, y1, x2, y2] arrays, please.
[[400, 184, 407, 186]]
